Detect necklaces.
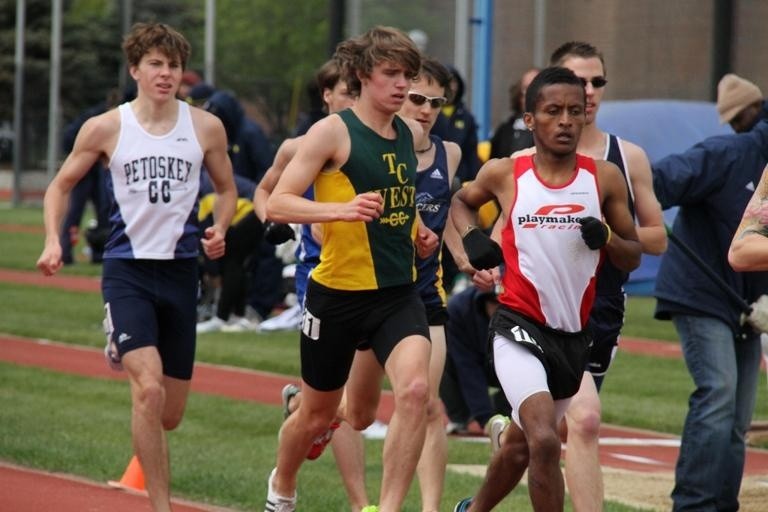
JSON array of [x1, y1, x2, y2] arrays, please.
[[416, 142, 433, 152]]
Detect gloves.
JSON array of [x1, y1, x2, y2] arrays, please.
[[578, 217, 610, 250], [740, 294, 768, 333], [459, 223, 503, 271]]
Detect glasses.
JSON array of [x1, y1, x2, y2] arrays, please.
[[407, 91, 448, 107], [577, 74, 607, 89]]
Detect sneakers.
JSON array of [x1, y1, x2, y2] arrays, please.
[[305, 419, 339, 460], [264, 468, 297, 511], [195, 301, 301, 335], [282, 385, 301, 420], [489, 415, 513, 452], [103, 318, 124, 372]]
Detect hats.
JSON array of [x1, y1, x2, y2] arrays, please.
[[716, 72, 765, 124]]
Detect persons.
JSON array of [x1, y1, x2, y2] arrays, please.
[[431, 66, 539, 435], [35, 21, 236, 512], [484, 42, 667, 512], [450, 67, 641, 512], [52, 113, 109, 266], [282, 56, 476, 512], [652, 73, 768, 512], [267, 28, 439, 512], [253, 57, 371, 510], [178, 69, 303, 335]]
